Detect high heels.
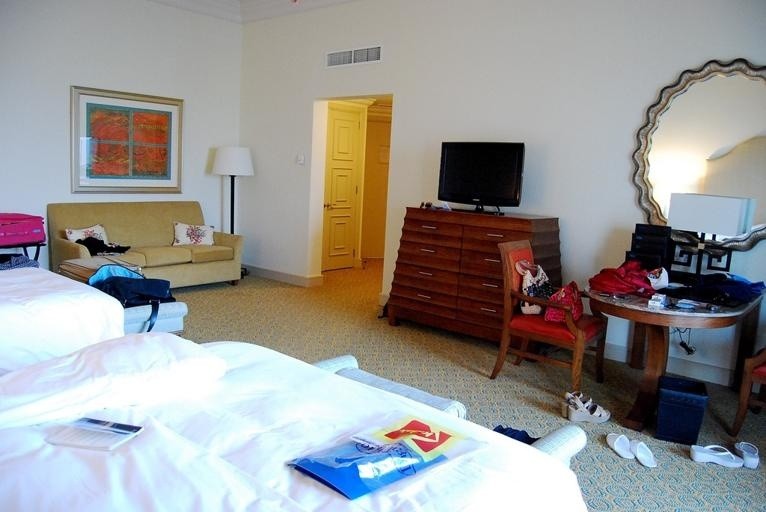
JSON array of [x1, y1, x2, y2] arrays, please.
[[561, 389, 593, 418], [567, 395, 611, 424]]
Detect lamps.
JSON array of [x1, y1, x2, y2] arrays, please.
[[211, 145, 256, 234]]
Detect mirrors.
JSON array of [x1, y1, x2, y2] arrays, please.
[[632, 57, 766, 259]]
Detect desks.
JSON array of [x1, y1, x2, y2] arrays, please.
[[584, 282, 764, 431]]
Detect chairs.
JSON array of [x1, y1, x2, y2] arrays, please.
[[489, 239, 609, 396], [729, 345, 766, 437]]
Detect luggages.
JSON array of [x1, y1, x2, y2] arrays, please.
[[0, 213, 46, 247]]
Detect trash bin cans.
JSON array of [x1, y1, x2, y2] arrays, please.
[[654, 374, 709, 446]]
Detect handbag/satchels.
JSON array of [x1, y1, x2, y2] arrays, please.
[[513, 256, 556, 317], [545, 279, 584, 323]]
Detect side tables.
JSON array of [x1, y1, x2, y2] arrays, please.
[[0, 243, 47, 261]]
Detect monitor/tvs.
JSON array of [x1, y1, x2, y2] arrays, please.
[[438, 142, 525, 216]]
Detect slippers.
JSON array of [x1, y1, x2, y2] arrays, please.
[[605, 431, 635, 458], [735, 442, 760, 470], [689, 444, 745, 468], [630, 438, 659, 468]]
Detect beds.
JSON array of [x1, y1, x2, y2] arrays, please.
[[1, 331, 589, 512], [0, 266, 126, 373]]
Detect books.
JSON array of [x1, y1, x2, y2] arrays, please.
[[45, 417, 145, 452]]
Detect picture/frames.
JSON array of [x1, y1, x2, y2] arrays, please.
[[69, 86, 184, 194]]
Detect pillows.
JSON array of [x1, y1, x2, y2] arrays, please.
[[172, 221, 215, 247], [65, 224, 109, 246]]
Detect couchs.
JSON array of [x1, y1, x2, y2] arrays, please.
[[47, 201, 245, 289]]
